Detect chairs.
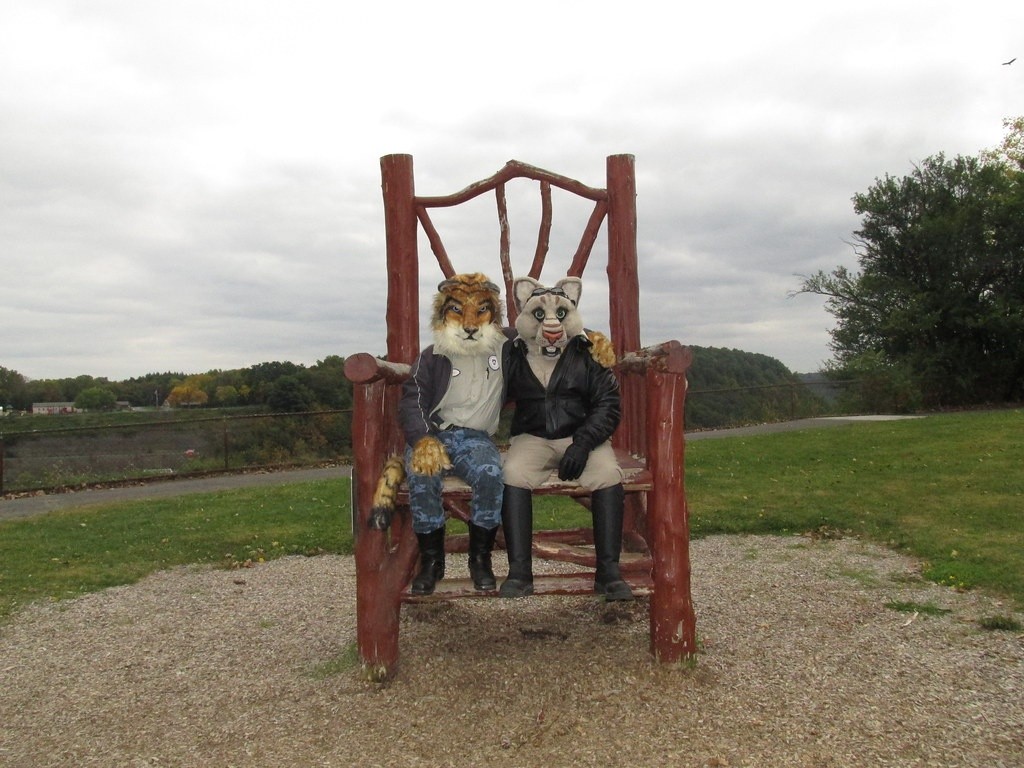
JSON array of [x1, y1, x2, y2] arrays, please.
[[342, 152, 694, 681]]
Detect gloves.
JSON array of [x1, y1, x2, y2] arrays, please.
[[558, 440, 589, 481]]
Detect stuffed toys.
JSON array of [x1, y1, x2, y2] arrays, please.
[[500, 276, 636, 602], [397, 273, 616, 598]]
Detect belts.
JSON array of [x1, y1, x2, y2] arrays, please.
[[431, 413, 463, 431]]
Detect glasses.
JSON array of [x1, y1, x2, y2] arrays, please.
[[526, 287, 575, 306]]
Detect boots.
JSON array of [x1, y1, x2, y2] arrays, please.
[[412, 523, 446, 594], [592, 483, 634, 601], [467, 518, 498, 590], [497, 482, 536, 598]]
